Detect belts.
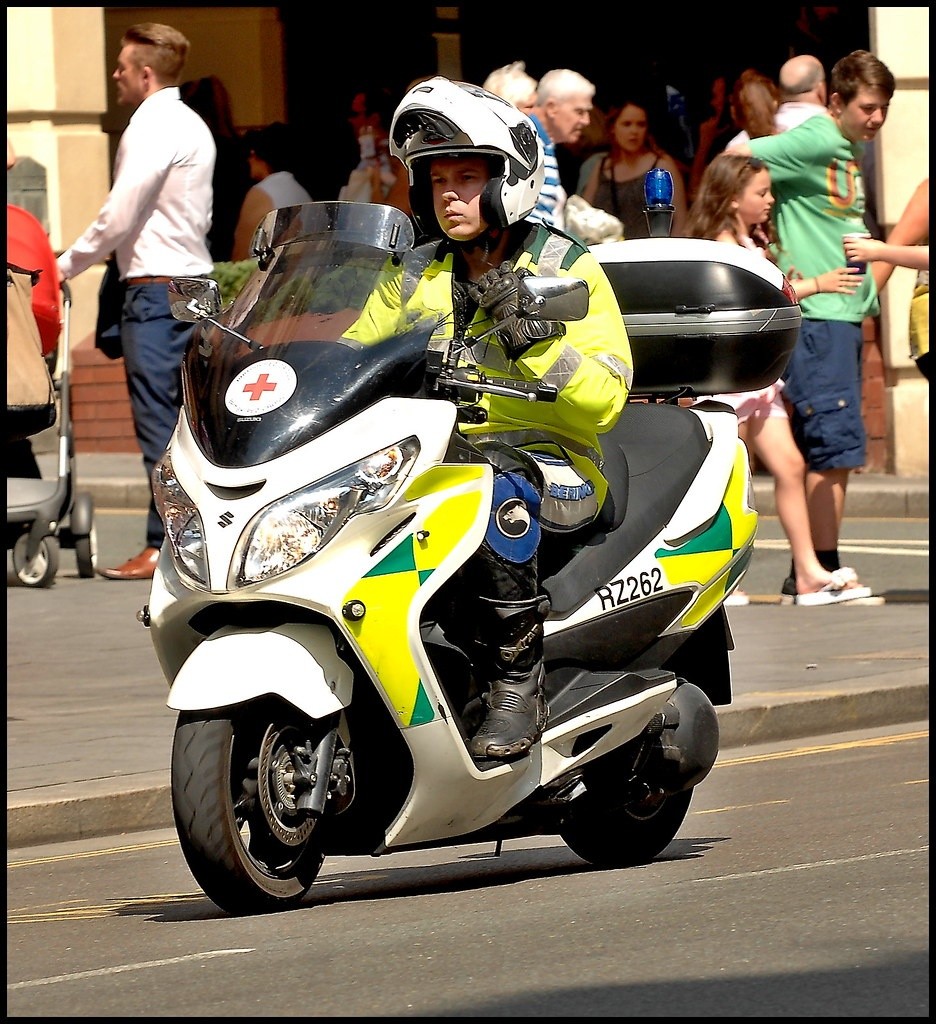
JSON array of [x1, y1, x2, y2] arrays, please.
[[128, 277, 171, 285]]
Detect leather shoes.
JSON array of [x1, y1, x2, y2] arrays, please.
[[98, 546, 159, 580]]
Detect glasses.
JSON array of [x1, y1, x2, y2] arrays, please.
[[736, 158, 766, 176]]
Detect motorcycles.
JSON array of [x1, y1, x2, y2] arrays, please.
[[136, 169, 805, 913]]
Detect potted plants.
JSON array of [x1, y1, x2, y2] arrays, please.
[[72, 253, 384, 457]]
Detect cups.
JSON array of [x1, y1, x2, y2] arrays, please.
[[842, 233, 872, 274]]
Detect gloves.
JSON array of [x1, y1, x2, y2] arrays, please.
[[468, 261, 566, 360]]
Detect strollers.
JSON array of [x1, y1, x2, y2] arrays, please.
[[9, 202, 98, 588]]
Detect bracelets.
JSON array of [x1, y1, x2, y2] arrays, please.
[[814, 277, 821, 293]]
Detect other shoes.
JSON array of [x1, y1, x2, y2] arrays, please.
[[782, 576, 886, 606]]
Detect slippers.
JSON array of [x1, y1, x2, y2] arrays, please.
[[796, 567, 872, 607], [722, 585, 750, 607]]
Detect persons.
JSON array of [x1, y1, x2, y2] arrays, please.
[[56, 18, 215, 580], [340, 75, 636, 760], [332, 51, 929, 608], [233, 127, 329, 260]]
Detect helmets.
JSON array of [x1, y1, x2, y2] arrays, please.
[[389, 75, 546, 227]]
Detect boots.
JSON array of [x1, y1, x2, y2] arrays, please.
[[470, 594, 551, 756]]
